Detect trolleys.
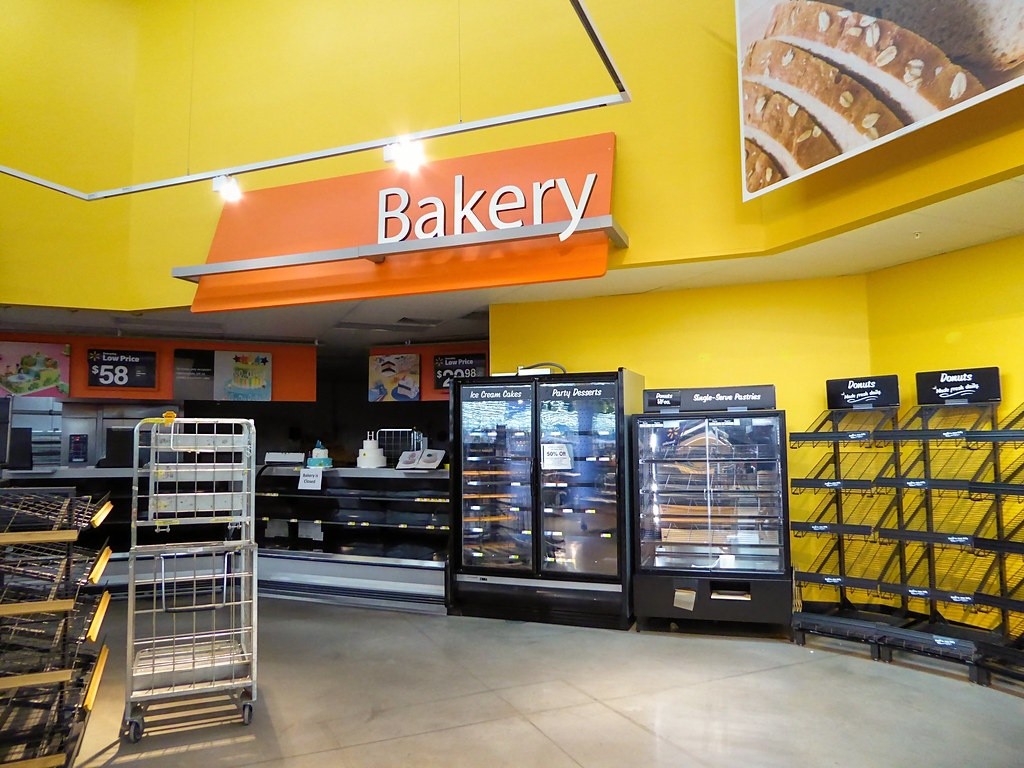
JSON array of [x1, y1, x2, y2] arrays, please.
[[121, 415, 264, 743]]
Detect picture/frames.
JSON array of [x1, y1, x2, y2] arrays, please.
[[735, 1, 1023, 204]]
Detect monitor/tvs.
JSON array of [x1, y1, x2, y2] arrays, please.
[[106, 427, 156, 468]]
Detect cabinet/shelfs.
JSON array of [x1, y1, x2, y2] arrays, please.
[[0, 486, 112, 766], [789, 403, 1023, 690], [255, 471, 450, 566], [124, 416, 256, 741], [14, 476, 232, 556]]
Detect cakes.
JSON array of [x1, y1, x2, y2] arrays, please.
[[232, 352, 270, 388], [397, 376, 419, 398], [356, 439, 386, 467], [373, 356, 401, 376], [306, 440, 332, 467]]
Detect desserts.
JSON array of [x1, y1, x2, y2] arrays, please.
[[422, 451, 437, 463], [402, 453, 416, 464]]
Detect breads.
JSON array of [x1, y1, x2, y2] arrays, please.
[[736, 0, 1023, 193]]
